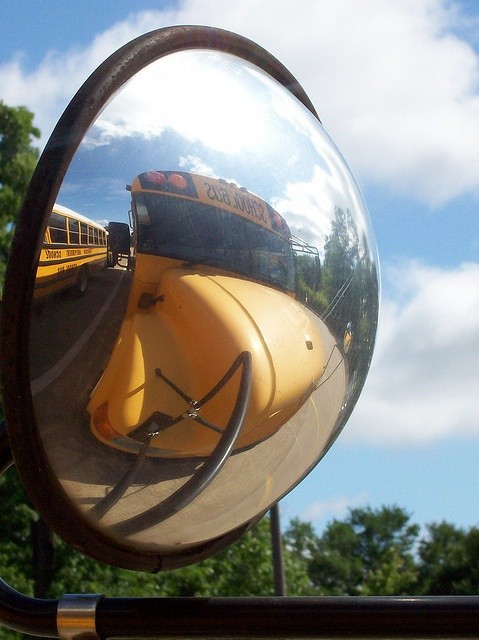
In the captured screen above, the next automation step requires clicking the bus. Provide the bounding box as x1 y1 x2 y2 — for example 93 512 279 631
29 202 108 308
84 168 335 464
